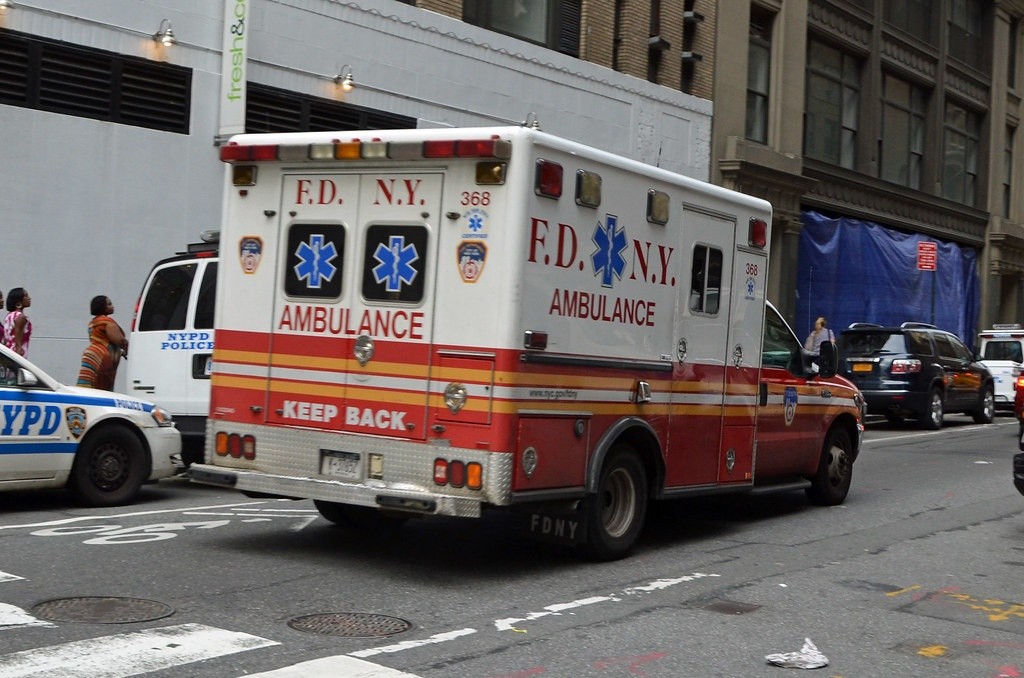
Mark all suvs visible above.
[834,321,995,432]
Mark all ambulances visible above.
[185,120,869,567]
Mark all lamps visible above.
[0,0,17,16]
[333,63,357,93]
[153,18,179,47]
[521,111,543,132]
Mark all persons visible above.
[0,287,32,385]
[806,317,835,352]
[76,296,129,392]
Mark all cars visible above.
[1,345,185,507]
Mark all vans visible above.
[112,231,220,465]
[976,323,1024,412]
